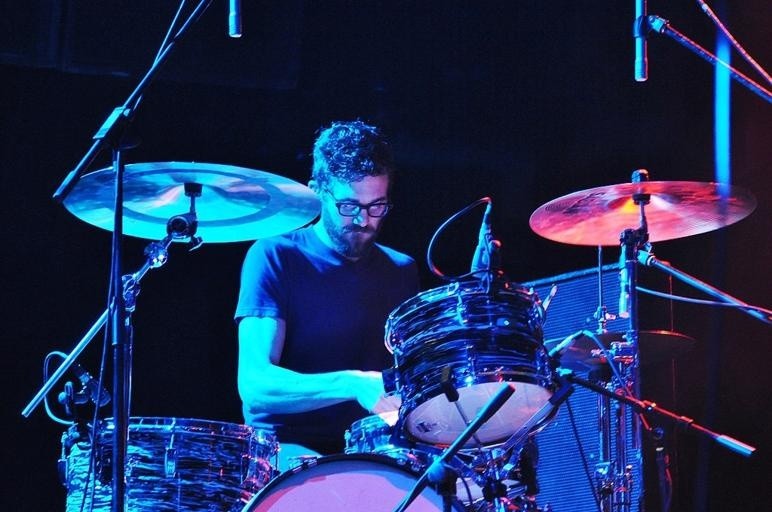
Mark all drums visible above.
[384,281,561,447]
[244,453,464,510]
[343,413,530,505]
[60,417,278,510]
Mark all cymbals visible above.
[530,181,757,246]
[539,331,696,359]
[62,161,322,244]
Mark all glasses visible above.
[321,185,394,218]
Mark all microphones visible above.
[548,330,584,359]
[470,204,492,279]
[634,0,649,83]
[229,0,243,38]
[68,358,111,407]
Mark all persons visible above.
[233,121,421,476]
